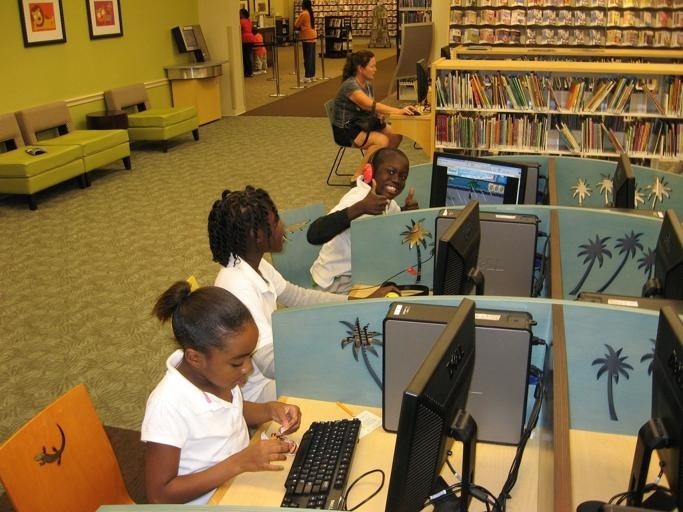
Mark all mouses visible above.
[403,110,420,115]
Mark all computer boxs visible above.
[503,160,539,205]
[433,209,538,297]
[381,302,531,446]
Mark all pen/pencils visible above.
[335,400,356,416]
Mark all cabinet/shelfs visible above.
[294,0,432,64]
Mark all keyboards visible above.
[280,419,360,511]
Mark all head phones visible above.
[380,281,430,297]
[364,148,390,183]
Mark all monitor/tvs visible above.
[430,151,528,207]
[433,200,484,295]
[640,208,683,300]
[384,298,503,511]
[172,25,199,52]
[440,46,452,58]
[605,152,636,209]
[415,58,431,112]
[577,305,683,512]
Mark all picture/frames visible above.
[18,0,67,48]
[86,0,123,41]
[254,0,270,16]
[240,0,249,17]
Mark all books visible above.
[435,57,683,175]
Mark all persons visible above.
[294,0,318,83]
[240,9,255,77]
[140,282,301,504]
[252,27,267,75]
[307,148,419,295]
[333,50,417,187]
[208,185,401,440]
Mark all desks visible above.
[389,103,430,158]
[86,111,129,129]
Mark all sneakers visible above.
[300,76,317,84]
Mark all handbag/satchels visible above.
[353,110,386,132]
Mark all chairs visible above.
[0,383,135,512]
[325,98,365,186]
[15,101,132,187]
[104,82,200,153]
[0,112,86,210]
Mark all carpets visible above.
[244,40,397,116]
